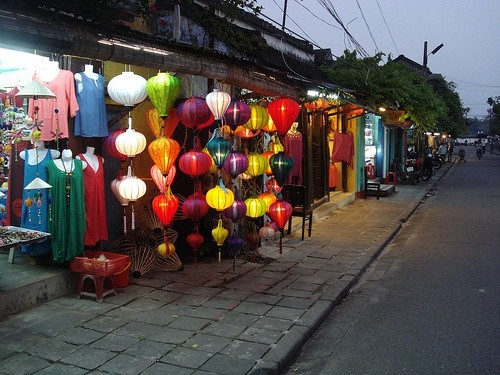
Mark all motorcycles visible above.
[476,148,483,160]
[403,150,446,185]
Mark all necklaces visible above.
[291,130,298,134]
[61,158,74,205]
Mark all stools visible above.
[385,172,397,186]
[77,272,117,302]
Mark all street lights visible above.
[423,41,444,67]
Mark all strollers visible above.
[458,149,466,163]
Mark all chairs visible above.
[363,165,380,201]
[283,184,313,241]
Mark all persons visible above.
[284,122,303,184]
[19,61,108,262]
[438,141,454,162]
[459,140,487,161]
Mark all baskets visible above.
[71,250,128,277]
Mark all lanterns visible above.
[304,98,328,111]
[103,72,300,258]
